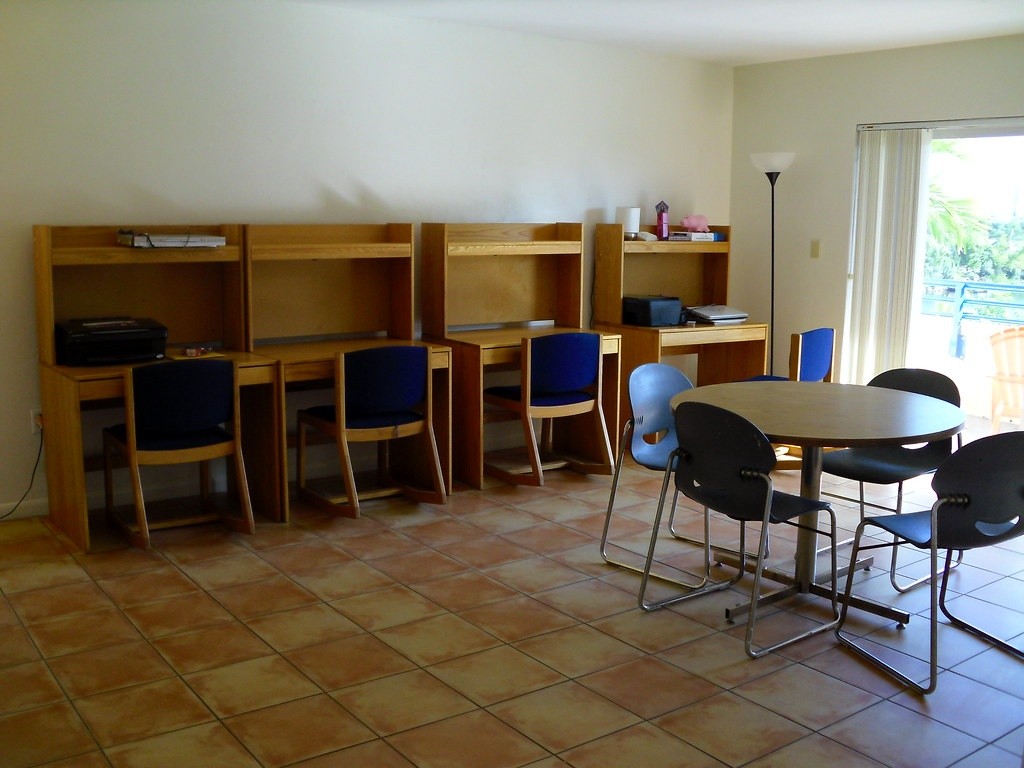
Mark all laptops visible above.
[117,233,227,248]
[685,305,749,320]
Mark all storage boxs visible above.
[624,295,682,327]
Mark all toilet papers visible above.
[615,206,640,233]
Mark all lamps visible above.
[748,151,798,374]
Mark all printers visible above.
[54,317,169,367]
[623,295,683,327]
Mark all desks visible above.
[664,381,966,629]
[31,221,770,556]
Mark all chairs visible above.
[772,327,844,470]
[601,363,1024,695]
[101,356,262,556]
[481,330,620,488]
[988,325,1024,437]
[295,344,447,522]
